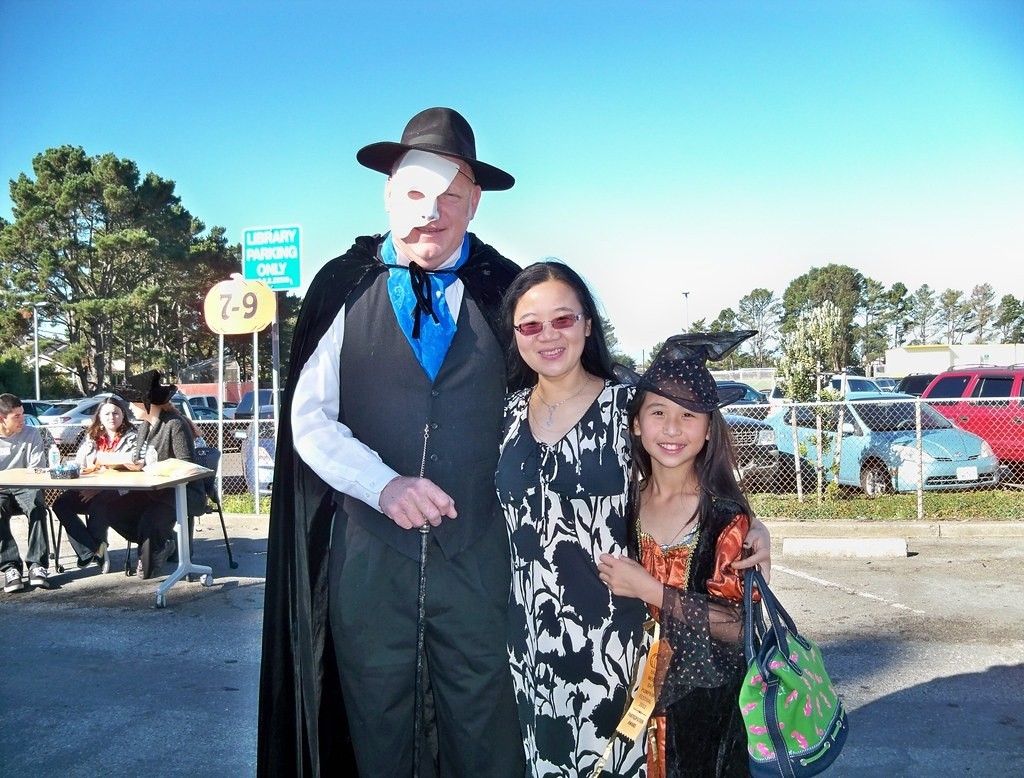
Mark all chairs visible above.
[54,513,132,576]
[11,504,62,572]
[125,447,239,579]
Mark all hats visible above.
[610,330,759,412]
[356,107,515,191]
[111,369,178,415]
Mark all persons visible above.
[257,102,523,778]
[102,368,207,579]
[53,397,138,573]
[598,331,760,778]
[495,262,770,778]
[0,394,50,592]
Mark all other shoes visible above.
[94,542,110,574]
[147,539,176,578]
[77,556,92,568]
[136,544,145,579]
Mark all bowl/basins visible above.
[47,468,80,479]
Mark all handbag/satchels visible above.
[739,566,848,778]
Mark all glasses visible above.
[512,315,582,336]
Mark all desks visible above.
[0,467,215,609]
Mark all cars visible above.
[759,363,1024,481]
[714,381,770,420]
[234,405,276,497]
[20,388,284,453]
[762,392,1000,500]
[718,408,780,492]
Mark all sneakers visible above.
[4,567,24,592]
[28,562,50,589]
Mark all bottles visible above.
[48,444,61,469]
[146,444,158,477]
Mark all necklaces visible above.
[536,372,591,423]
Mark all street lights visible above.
[681,291,691,346]
[22,301,51,400]
